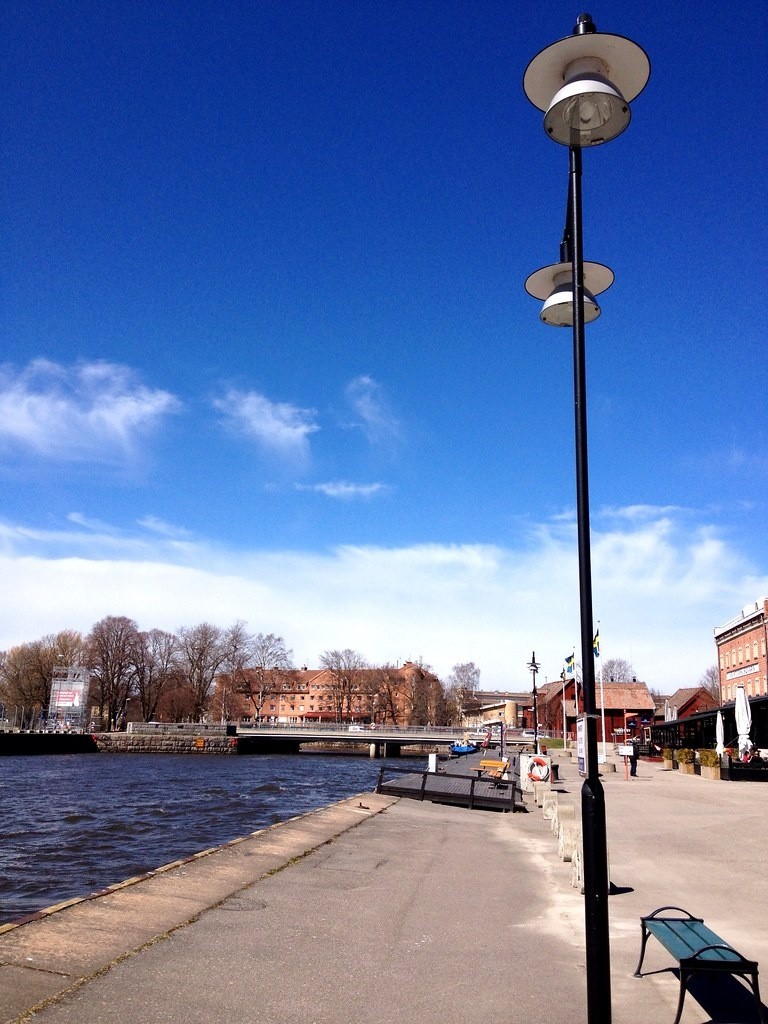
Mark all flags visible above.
[565,653,575,672]
[593,629,600,657]
[560,670,566,683]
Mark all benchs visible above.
[634,906,762,1024]
[470,768,502,779]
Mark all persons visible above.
[0,700,8,721]
[742,750,764,767]
[628,740,640,777]
[116,708,128,731]
[18,707,34,729]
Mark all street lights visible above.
[527,651,539,754]
[522,11,653,1024]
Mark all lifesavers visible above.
[526,757,550,780]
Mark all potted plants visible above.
[698,750,721,779]
[675,748,696,774]
[662,748,676,769]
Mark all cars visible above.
[521,730,551,740]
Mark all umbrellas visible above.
[735,686,752,762]
[665,698,677,722]
[716,711,724,766]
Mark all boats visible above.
[449,740,477,759]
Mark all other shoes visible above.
[632,774,637,777]
